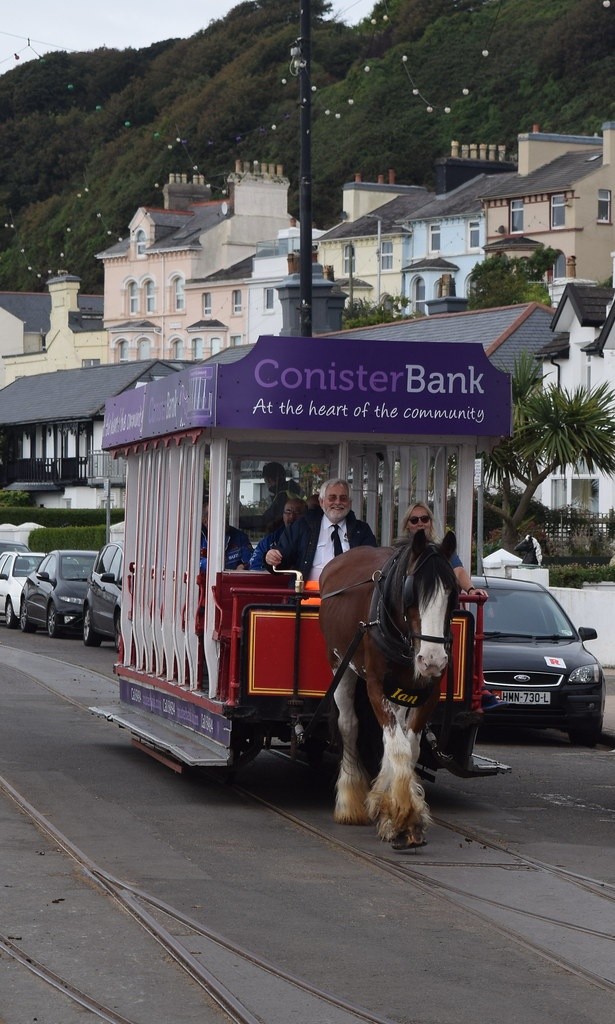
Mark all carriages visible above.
[83,334,515,849]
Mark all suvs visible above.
[80,542,125,656]
[0,540,39,572]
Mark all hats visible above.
[259,462,286,479]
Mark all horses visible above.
[317,528,461,851]
[513,534,543,566]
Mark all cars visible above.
[471,575,606,748]
[17,549,101,639]
[0,550,80,629]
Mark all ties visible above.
[330,525,343,557]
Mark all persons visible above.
[403,500,489,598]
[261,478,379,605]
[200,462,319,571]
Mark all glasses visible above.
[324,495,350,501]
[408,515,430,524]
